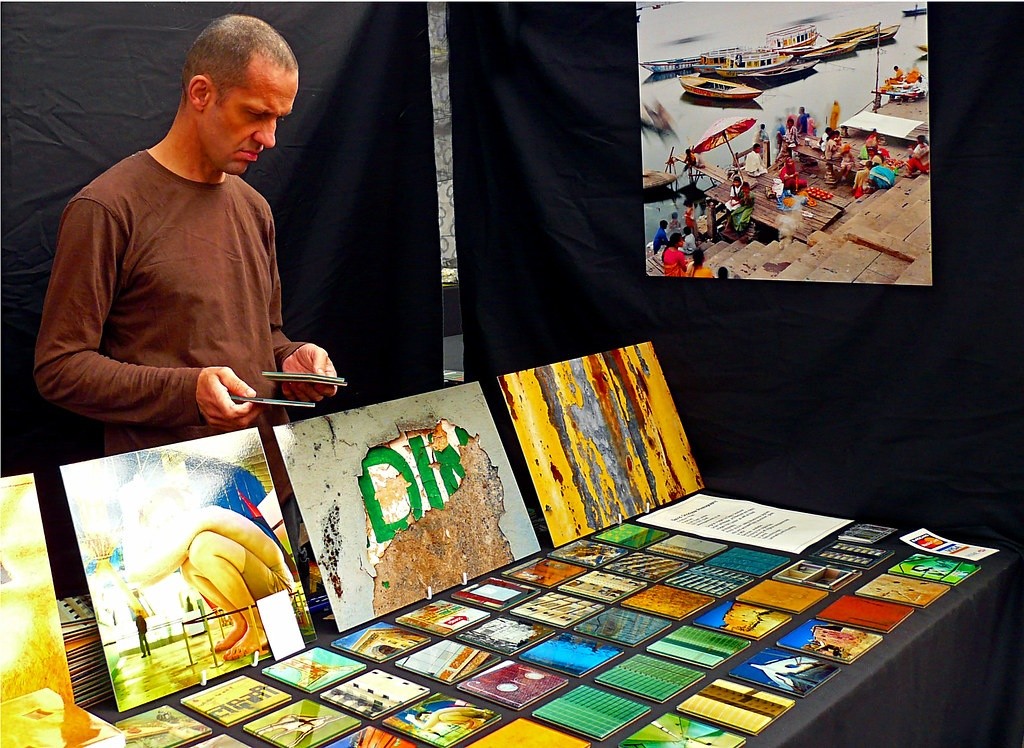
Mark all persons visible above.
[653,65,930,278]
[32,13,347,566]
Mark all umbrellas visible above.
[692,116,758,182]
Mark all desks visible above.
[0,492,1024,748]
